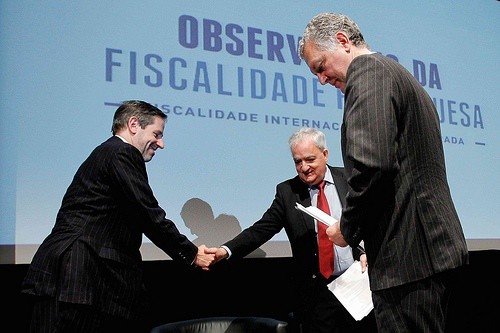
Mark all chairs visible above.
[151,317,288,333]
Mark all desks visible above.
[0,238,500,333]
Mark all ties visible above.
[317,181,335,280]
